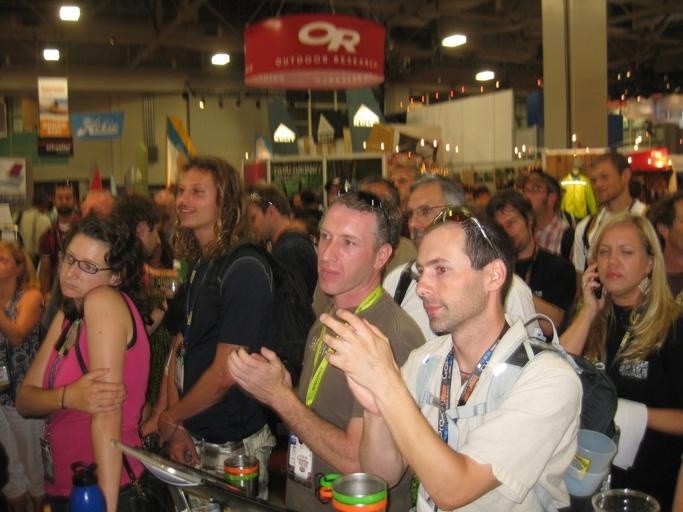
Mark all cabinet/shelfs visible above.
[620,123,677,152]
[452,158,540,196]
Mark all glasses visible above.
[60,250,112,274]
[428,205,500,258]
[356,188,391,243]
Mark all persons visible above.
[0,152,683,512]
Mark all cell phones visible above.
[594,267,603,299]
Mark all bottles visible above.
[66,462,106,511]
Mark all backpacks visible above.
[414,312,618,432]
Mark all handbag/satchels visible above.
[117,467,175,511]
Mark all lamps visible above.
[436,16,467,49]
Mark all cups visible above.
[224,455,259,496]
[316,471,388,512]
[567,429,660,511]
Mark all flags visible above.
[163,113,196,189]
